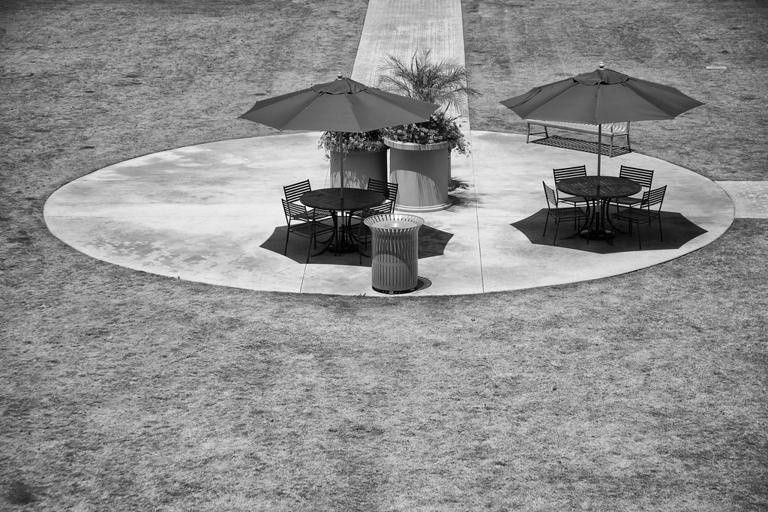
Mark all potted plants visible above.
[319,50,484,211]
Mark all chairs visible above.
[542,164,666,250]
[280,177,397,264]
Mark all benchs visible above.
[527,117,632,158]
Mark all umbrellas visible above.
[498,62,705,232]
[237,71,441,247]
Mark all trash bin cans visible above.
[363,214,424,294]
